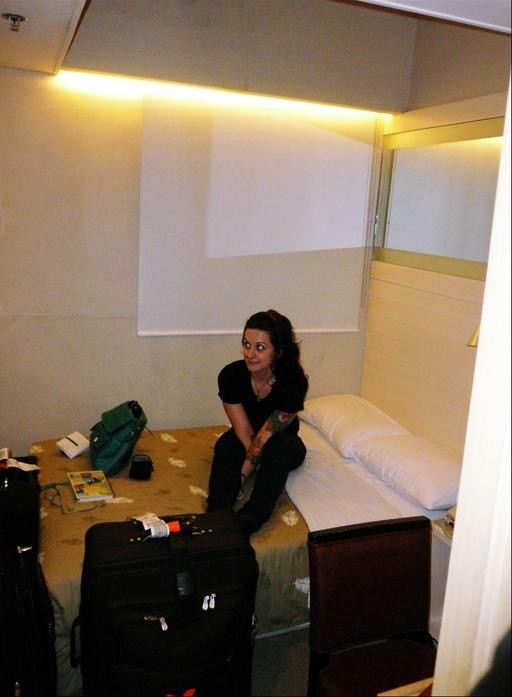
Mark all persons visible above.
[206,309,310,532]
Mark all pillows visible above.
[297,392,412,460]
[353,429,459,511]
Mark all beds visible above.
[28,418,451,697]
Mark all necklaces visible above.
[250,376,272,403]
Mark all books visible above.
[65,470,114,502]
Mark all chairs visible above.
[306,516,436,697]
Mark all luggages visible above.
[69,510,261,697]
[0,447,40,551]
[0,545,57,697]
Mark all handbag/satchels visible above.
[88,400,146,479]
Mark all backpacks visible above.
[89,400,147,477]
